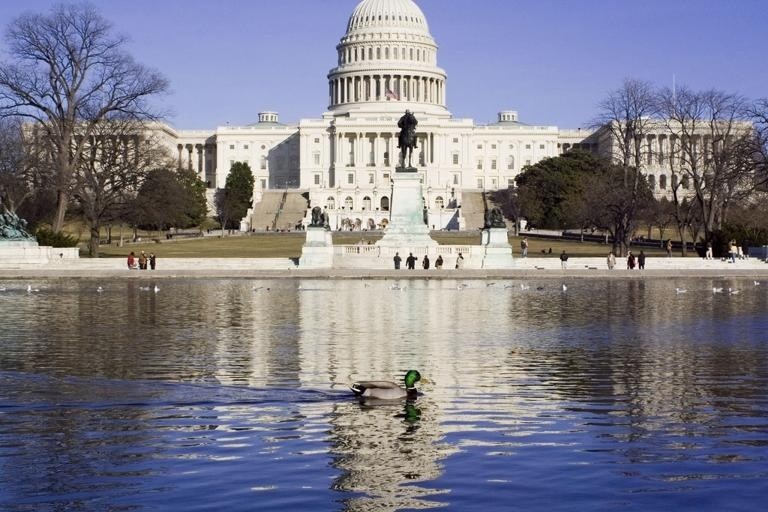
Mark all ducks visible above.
[350,370,431,400]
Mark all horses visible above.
[398,112,415,169]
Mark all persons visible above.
[521,237,528,257]
[423,255,429,269]
[560,250,568,269]
[128,250,155,269]
[397,109,418,148]
[455,253,464,269]
[627,250,645,269]
[394,252,401,269]
[406,253,417,269]
[728,239,743,262]
[666,239,672,257]
[435,255,443,269]
[607,252,616,269]
[704,239,713,260]
